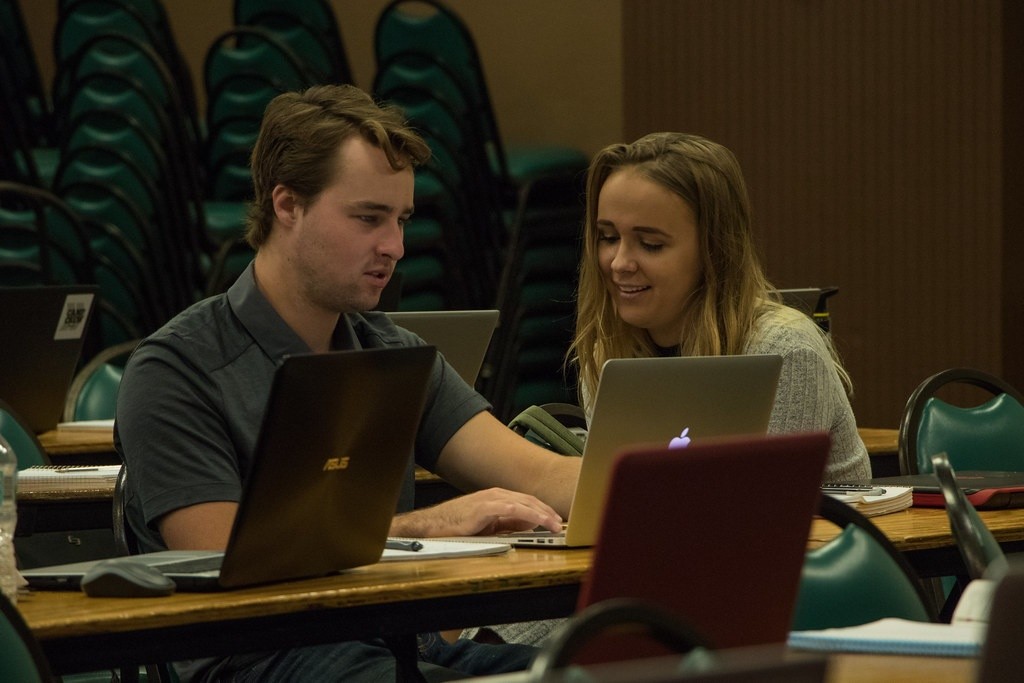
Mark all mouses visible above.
[80,559,176,598]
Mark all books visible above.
[58,419,114,432]
[379,537,512,561]
[17,465,121,490]
[788,617,988,659]
[815,484,914,518]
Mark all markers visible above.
[384,539,425,551]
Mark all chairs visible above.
[789,368,1024,635]
[111,461,181,683]
[0,0,603,471]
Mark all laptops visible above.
[21,288,832,664]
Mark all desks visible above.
[857,425,900,481]
[9,495,1024,683]
[36,421,117,459]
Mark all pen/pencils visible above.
[819,482,888,497]
[54,468,99,473]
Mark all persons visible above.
[114,86,583,683]
[459,133,872,647]
